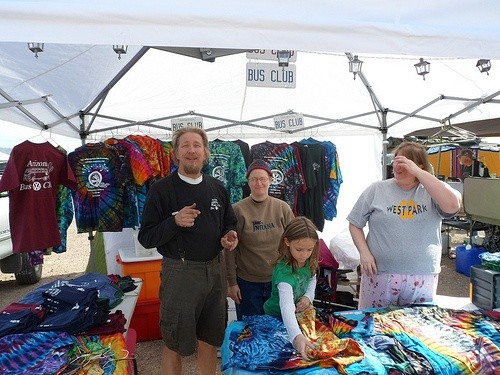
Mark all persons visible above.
[139,127,239,375]
[458,149,492,183]
[229,160,295,323]
[263,217,319,361]
[345,141,462,312]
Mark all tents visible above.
[0,0,500,252]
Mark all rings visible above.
[299,350,304,353]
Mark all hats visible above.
[245,158,273,179]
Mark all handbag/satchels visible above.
[317,238,339,270]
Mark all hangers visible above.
[420,116,478,147]
[26,123,332,145]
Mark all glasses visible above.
[248,176,270,183]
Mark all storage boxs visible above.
[116,246,163,345]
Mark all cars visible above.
[0,160,42,285]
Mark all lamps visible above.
[27,43,493,87]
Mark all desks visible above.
[107,277,144,375]
